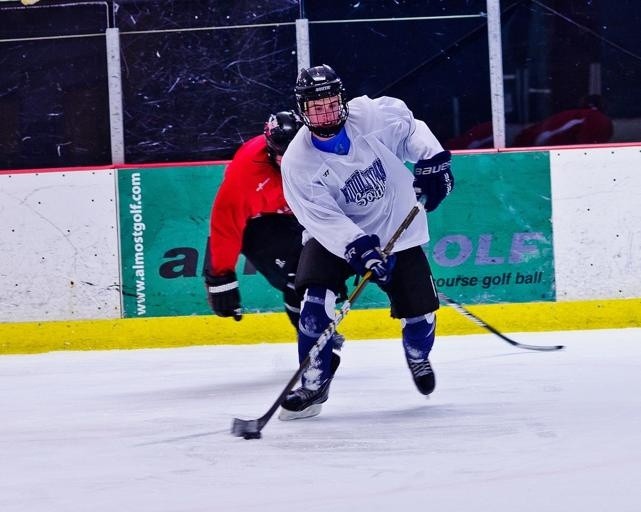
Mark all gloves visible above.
[343,234,396,286]
[412,150,454,213]
[205,269,243,322]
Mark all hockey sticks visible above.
[231,195,428,436]
[435,290,567,350]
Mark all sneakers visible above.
[280,377,332,411]
[407,355,436,395]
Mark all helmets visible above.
[295,62,348,138]
[264,110,304,152]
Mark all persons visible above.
[278,64,454,422]
[204,110,345,359]
